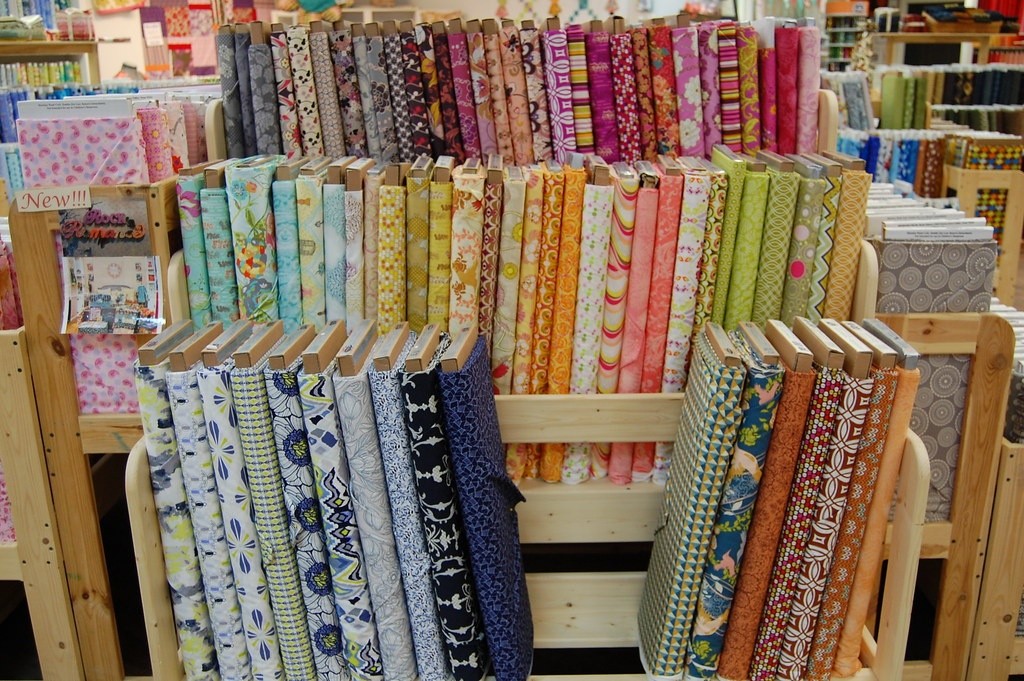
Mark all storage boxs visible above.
[921,11,1004,33]
